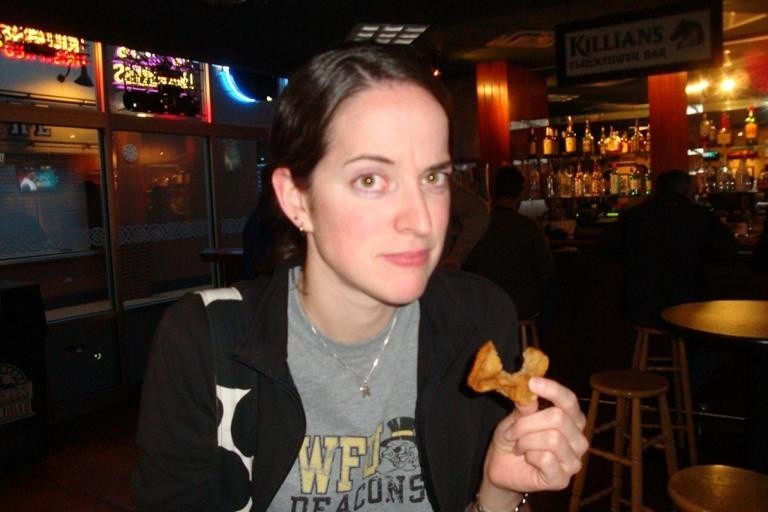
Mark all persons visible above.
[19,167,37,192]
[604,170,740,422]
[462,164,549,318]
[130,42,589,512]
[440,179,492,269]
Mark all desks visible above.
[661,298,768,339]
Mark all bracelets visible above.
[475,491,529,512]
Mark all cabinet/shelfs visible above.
[511,104,768,233]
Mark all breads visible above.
[465,340,549,408]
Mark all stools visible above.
[570,369,679,511]
[625,327,698,467]
[517,320,540,351]
[668,464,767,511]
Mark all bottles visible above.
[519,117,650,196]
[694,107,768,193]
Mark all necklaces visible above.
[293,281,403,397]
